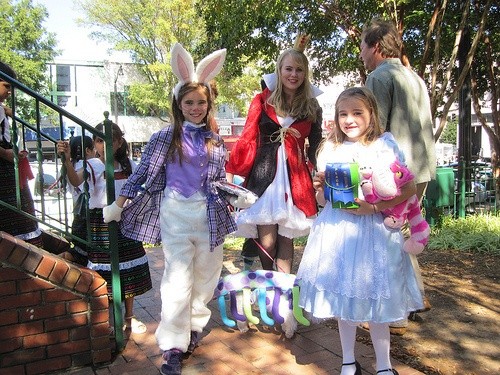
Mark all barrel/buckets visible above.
[425,167,454,208]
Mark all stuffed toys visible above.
[357,133,430,253]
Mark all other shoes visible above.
[360,322,406,335]
[342,359,362,375]
[416,295,431,313]
[377,368,399,375]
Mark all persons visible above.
[103,81,257,375]
[57,121,153,334]
[360,20,438,337]
[68,134,95,264]
[226,51,323,338]
[0,61,45,250]
[295,87,426,375]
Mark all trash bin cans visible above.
[423,167,456,228]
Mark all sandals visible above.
[125,315,146,333]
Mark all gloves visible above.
[103,201,123,223]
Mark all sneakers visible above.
[160,348,183,375]
[187,331,197,352]
